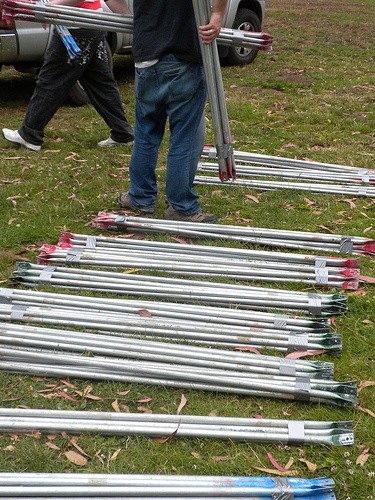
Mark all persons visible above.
[2,0,135,151]
[103,0,229,224]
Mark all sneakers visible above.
[117,191,153,214]
[98,134,135,148]
[163,205,216,223]
[2,127,41,151]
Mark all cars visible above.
[0,0,264,105]
